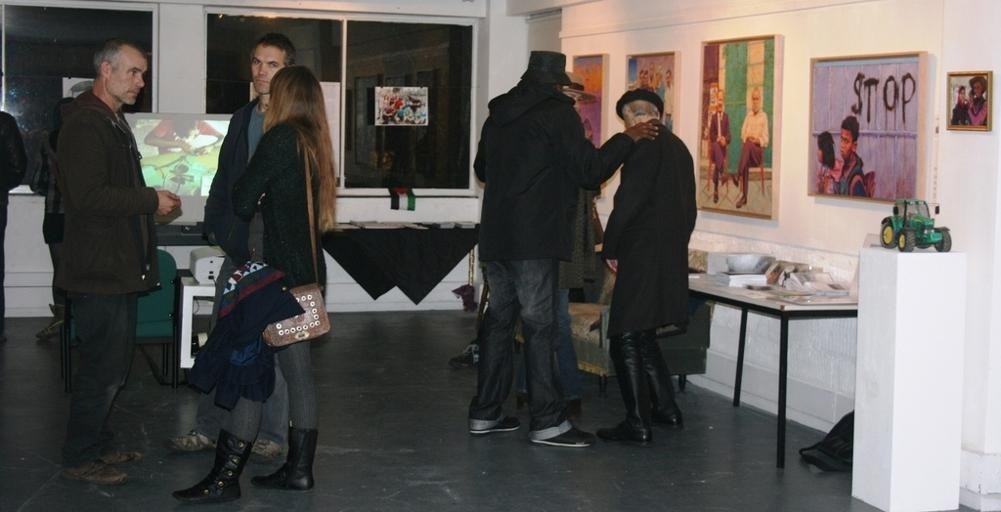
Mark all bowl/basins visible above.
[725,252,763,274]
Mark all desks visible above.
[43,221,482,308]
[684,273,861,467]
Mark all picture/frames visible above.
[572,53,609,199]
[947,71,992,132]
[627,50,683,134]
[807,50,940,209]
[695,34,785,219]
[375,86,428,126]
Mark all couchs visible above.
[479,240,711,400]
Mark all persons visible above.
[559,70,607,303]
[710,90,731,203]
[56,38,182,485]
[817,131,836,194]
[837,116,867,197]
[951,87,971,125]
[175,31,297,458]
[173,66,336,503]
[967,77,987,126]
[0,111,28,344]
[597,88,698,442]
[470,49,596,449]
[733,88,769,208]
[382,94,403,124]
[30,96,74,339]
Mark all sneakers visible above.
[98,450,142,464]
[449,337,481,368]
[62,459,127,485]
[169,431,212,453]
[530,426,594,448]
[251,437,281,464]
[471,416,519,434]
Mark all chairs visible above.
[63,249,179,392]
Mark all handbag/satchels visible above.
[248,123,330,348]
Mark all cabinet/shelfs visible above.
[183,275,216,368]
[855,250,963,512]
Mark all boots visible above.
[251,424,317,490]
[171,428,253,502]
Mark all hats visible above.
[616,88,663,119]
[519,49,572,85]
[562,72,596,102]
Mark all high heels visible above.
[517,394,529,409]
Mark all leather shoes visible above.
[596,421,649,444]
[651,406,683,425]
